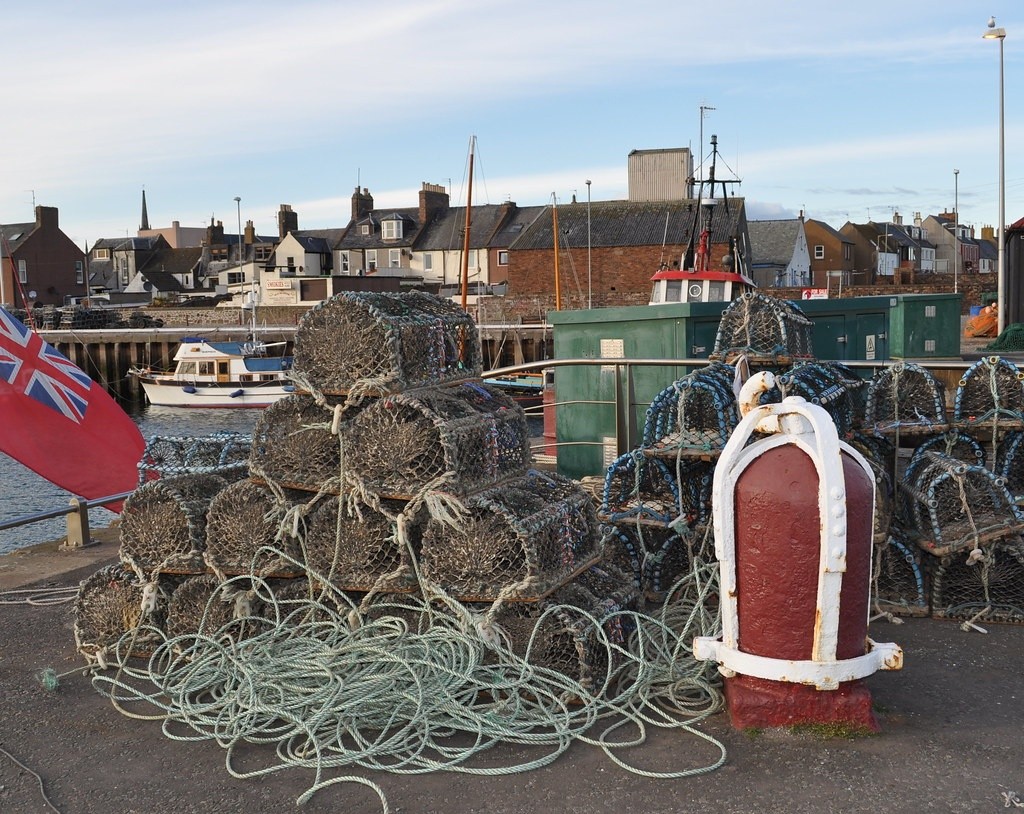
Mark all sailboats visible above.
[439,137,587,411]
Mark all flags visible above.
[0,306,160,515]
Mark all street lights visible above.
[942,223,949,259]
[877,234,894,275]
[235,196,243,322]
[885,222,895,279]
[954,169,959,291]
[981,28,1007,339]
[62,295,71,306]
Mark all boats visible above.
[124,280,297,409]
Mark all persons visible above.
[33,298,43,328]
